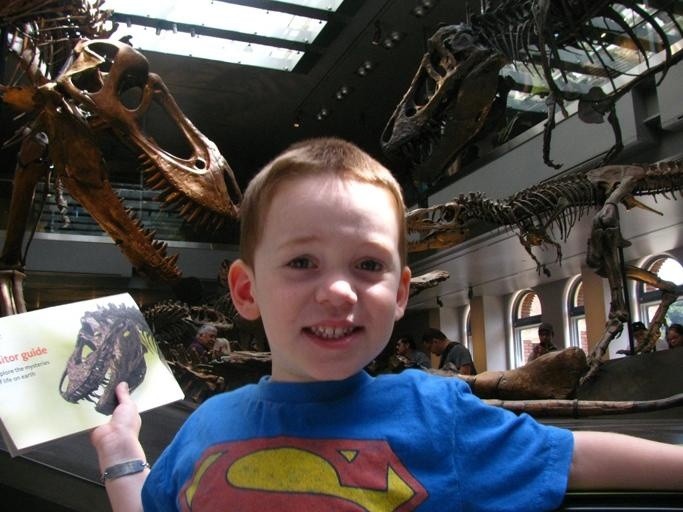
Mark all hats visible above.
[539,323,552,334]
[632,322,647,331]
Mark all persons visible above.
[528,322,561,364]
[421,328,478,375]
[393,335,432,370]
[667,323,683,348]
[187,324,218,356]
[631,321,657,353]
[90,139,683,512]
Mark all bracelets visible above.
[99,459,151,484]
